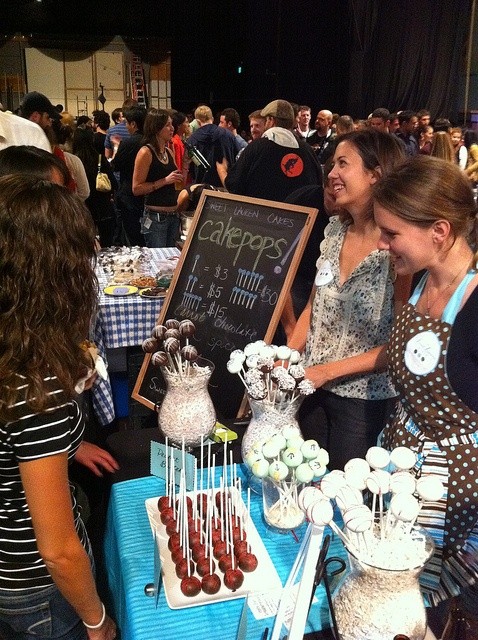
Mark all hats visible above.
[19,91,63,119]
[260,100,294,120]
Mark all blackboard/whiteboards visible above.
[130,188,319,418]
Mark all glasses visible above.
[91,120,99,123]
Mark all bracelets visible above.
[182,164,190,172]
[82,602,107,629]
[152,181,157,192]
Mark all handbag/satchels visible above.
[96,154,112,193]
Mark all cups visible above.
[262,472,308,532]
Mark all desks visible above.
[100,463,430,640]
[92,246,182,429]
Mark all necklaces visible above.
[421,247,474,320]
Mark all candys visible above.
[243,423,329,523]
[155,430,262,600]
[223,337,315,409]
[139,314,201,392]
[297,445,444,564]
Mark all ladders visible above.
[130,53,146,109]
[77,96,88,116]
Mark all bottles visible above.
[181,138,213,176]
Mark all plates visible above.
[139,286,172,299]
[104,285,138,297]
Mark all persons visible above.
[72,124,118,246]
[52,120,91,201]
[0,145,70,186]
[398,110,449,159]
[17,91,63,130]
[372,155,477,626]
[132,108,193,248]
[60,111,74,127]
[75,115,92,128]
[330,114,340,129]
[44,125,75,193]
[112,108,126,125]
[306,109,338,166]
[0,109,52,154]
[295,105,312,143]
[289,103,303,138]
[241,129,247,140]
[104,99,141,244]
[278,162,354,354]
[90,109,111,156]
[272,129,415,473]
[235,109,267,161]
[177,183,214,212]
[171,112,191,189]
[355,108,398,140]
[185,104,243,187]
[109,104,147,247]
[165,108,178,122]
[184,119,200,137]
[187,113,193,123]
[217,108,249,148]
[334,115,355,143]
[449,127,478,183]
[0,172,122,640]
[215,99,323,203]
